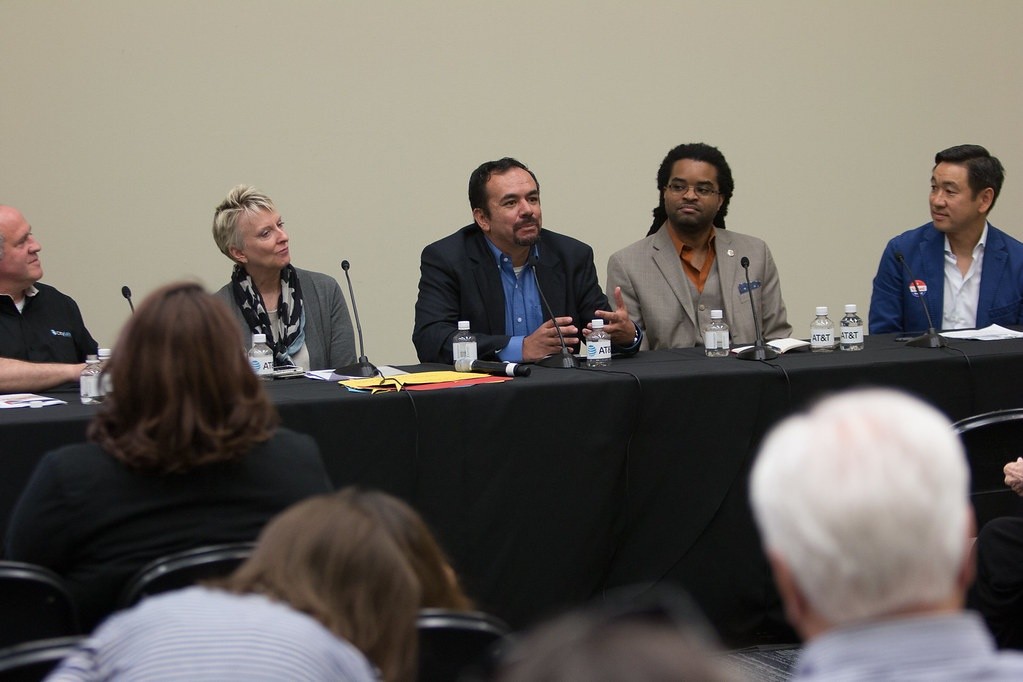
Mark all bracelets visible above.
[634,329,638,345]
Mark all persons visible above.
[411,158,643,363]
[211,186,357,372]
[499,384,1023,682]
[0,279,469,682]
[605,141,792,350]
[869,144,1023,336]
[0,204,100,393]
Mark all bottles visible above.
[97,349,112,392]
[247,333,274,381]
[453,320,477,365]
[586,319,612,367]
[705,309,729,357]
[840,304,864,351]
[80,355,106,405]
[810,307,834,353]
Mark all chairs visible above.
[121,542,257,611]
[414,608,519,682]
[0,562,85,642]
[0,637,85,682]
[949,408,1023,533]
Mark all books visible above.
[0,394,69,410]
[731,337,810,354]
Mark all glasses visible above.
[665,181,721,199]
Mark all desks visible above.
[0,328,1023,644]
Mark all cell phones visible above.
[895,332,926,342]
[273,367,304,376]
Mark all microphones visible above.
[528,256,580,368]
[333,260,378,377]
[455,357,531,377]
[121,285,135,314]
[735,257,779,361]
[894,251,949,349]
